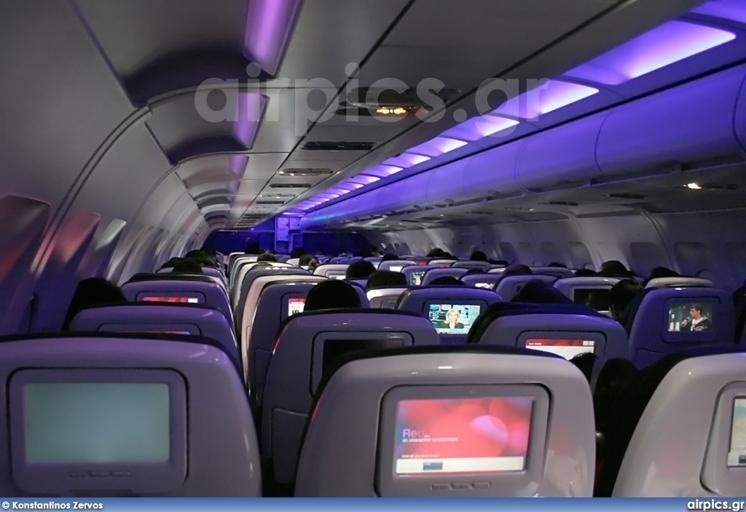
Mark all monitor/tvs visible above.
[7,252,746,497]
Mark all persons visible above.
[63,239,746,498]
